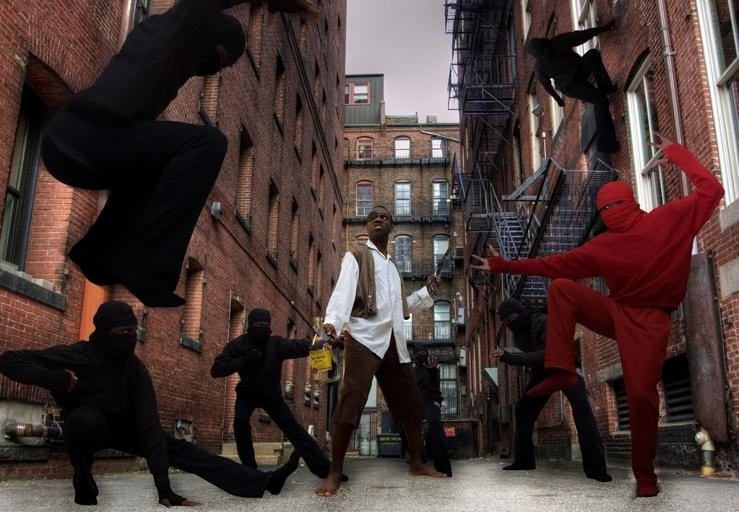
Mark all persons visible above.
[316,205,451,497]
[0,300,302,510]
[208,308,350,486]
[487,297,613,485]
[465,132,725,498]
[40,1,249,309]
[524,15,624,155]
[405,346,453,480]
